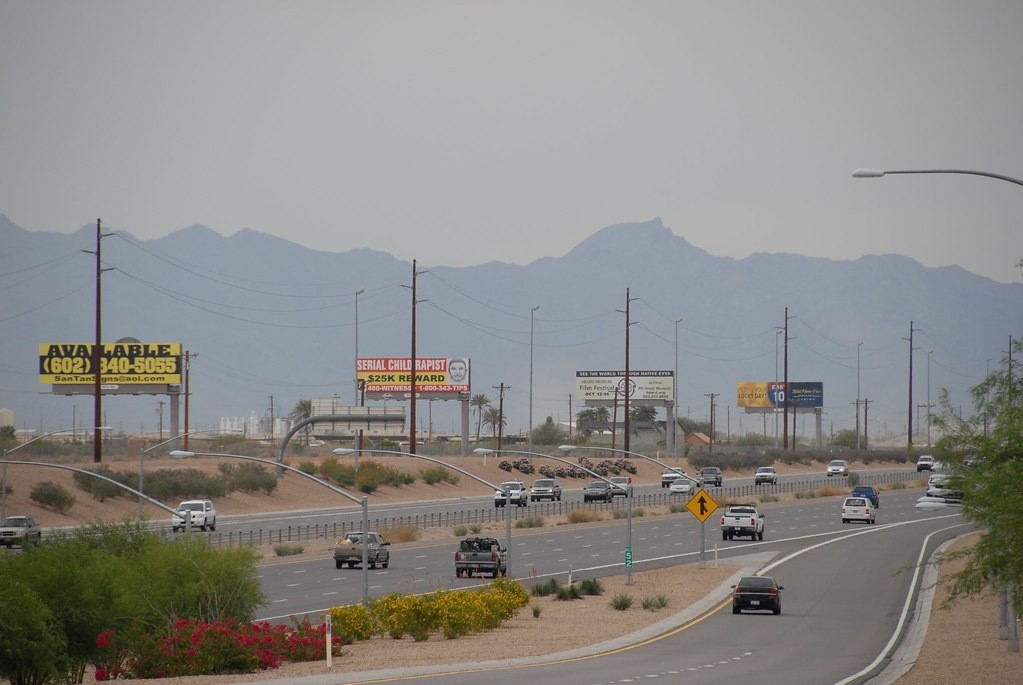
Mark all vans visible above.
[843,485,879,525]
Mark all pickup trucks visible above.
[332,532,391,569]
[721,504,765,541]
[454,538,509,580]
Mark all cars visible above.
[669,479,697,496]
[755,467,778,485]
[827,459,849,476]
[731,576,784,615]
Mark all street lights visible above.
[171,450,368,606]
[333,448,510,579]
[354,288,993,451]
[0,426,244,518]
[559,445,706,567]
[471,449,633,585]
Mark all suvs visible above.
[0,515,41,550]
[495,479,562,508]
[916,455,977,500]
[701,467,722,487]
[661,468,687,487]
[583,477,633,503]
[171,500,217,533]
